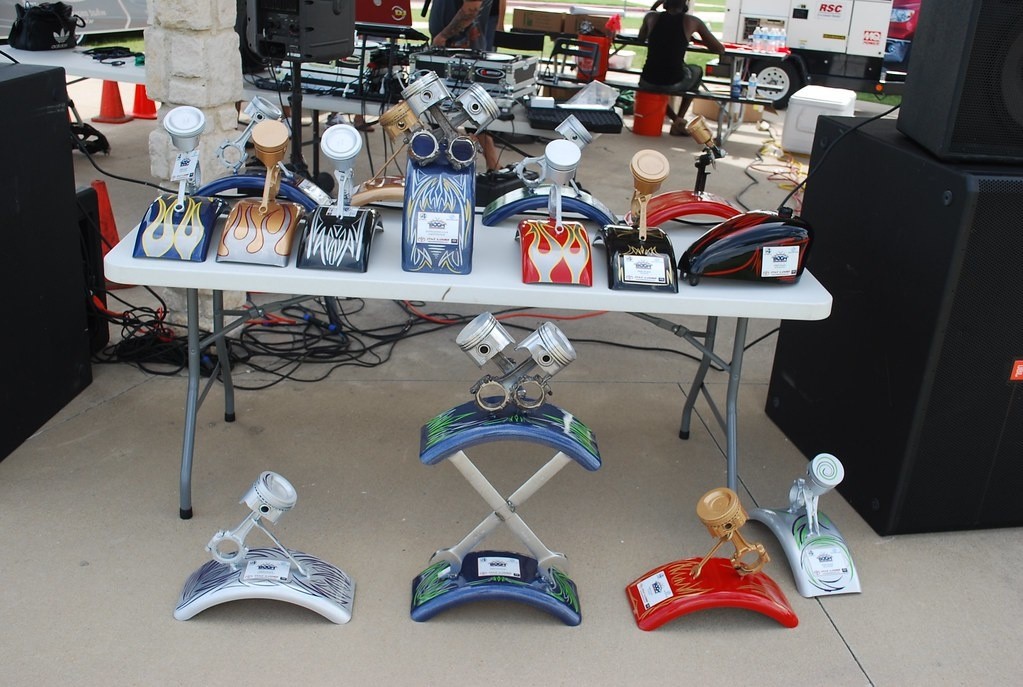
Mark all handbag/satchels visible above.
[8,1,85,52]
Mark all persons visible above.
[637,1,724,137]
[429,0,506,173]
[485,1,505,53]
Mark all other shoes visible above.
[669,119,690,137]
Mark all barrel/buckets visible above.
[576,34,610,83]
[632,91,670,135]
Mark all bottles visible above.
[751,27,787,53]
[731,72,741,99]
[746,73,757,100]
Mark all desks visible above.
[101,204,833,527]
[0,1,790,180]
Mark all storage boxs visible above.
[779,85,857,155]
